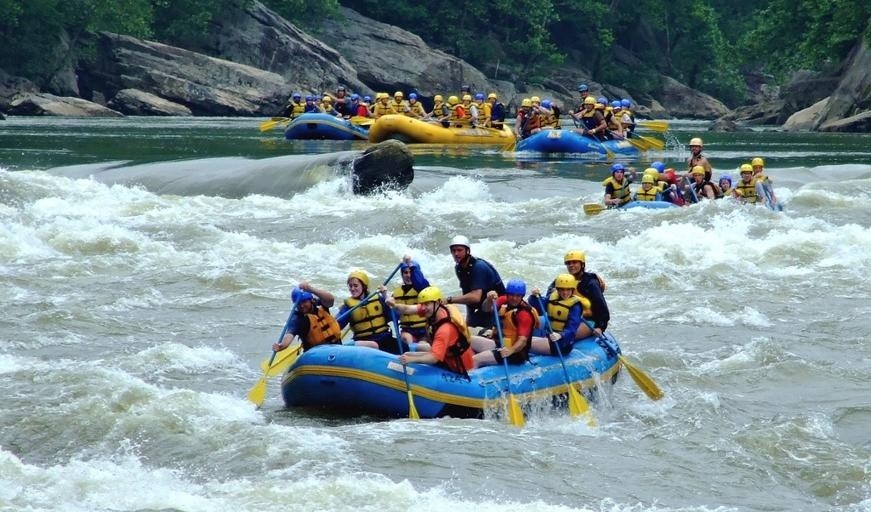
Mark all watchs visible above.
[446,295,453,303]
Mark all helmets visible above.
[363,96,371,102]
[611,100,621,107]
[621,99,630,107]
[555,273,576,290]
[460,85,469,92]
[521,99,531,106]
[530,96,539,102]
[641,174,653,184]
[474,93,483,99]
[739,164,752,176]
[408,93,416,98]
[461,95,470,100]
[447,96,457,104]
[347,271,368,287]
[541,100,549,108]
[719,176,731,187]
[643,168,658,182]
[487,93,496,99]
[375,93,380,99]
[290,287,313,303]
[304,96,312,100]
[401,260,420,272]
[564,250,584,263]
[314,95,321,100]
[689,138,702,147]
[418,286,443,304]
[612,163,623,176]
[692,166,704,176]
[292,93,300,98]
[597,98,607,106]
[578,84,587,92]
[350,94,358,100]
[751,158,762,166]
[322,96,330,102]
[448,235,469,249]
[651,161,663,173]
[380,92,389,100]
[506,279,526,295]
[584,96,594,104]
[394,91,403,97]
[433,95,442,102]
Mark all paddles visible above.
[388,304,420,418]
[491,298,524,427]
[571,112,616,159]
[605,127,650,152]
[623,127,666,151]
[470,122,500,136]
[505,107,533,155]
[351,115,376,125]
[262,290,382,378]
[584,204,625,216]
[634,121,668,133]
[535,293,590,418]
[248,290,305,407]
[260,117,290,132]
[580,315,664,402]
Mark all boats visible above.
[613,195,673,211]
[510,127,637,155]
[369,115,510,142]
[282,110,364,138]
[280,317,622,420]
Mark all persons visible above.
[304,95,322,113]
[663,170,721,206]
[360,96,374,118]
[272,280,343,353]
[323,86,351,117]
[389,253,434,353]
[651,161,666,175]
[446,96,467,128]
[370,93,383,114]
[405,92,430,121]
[550,102,561,130]
[573,96,608,140]
[285,93,308,124]
[719,174,739,202]
[470,279,542,371]
[736,163,769,207]
[602,163,637,208]
[598,97,623,140]
[336,271,398,353]
[532,96,551,132]
[542,99,555,130]
[462,94,478,129]
[634,174,679,204]
[374,93,398,121]
[443,234,505,330]
[575,84,591,114]
[314,93,323,114]
[475,93,491,129]
[350,93,369,117]
[320,96,343,117]
[488,93,506,130]
[528,273,592,356]
[680,136,713,184]
[385,285,476,373]
[611,100,634,137]
[680,165,716,204]
[514,98,542,140]
[752,158,778,211]
[391,91,421,119]
[644,168,679,203]
[621,98,636,132]
[546,249,613,334]
[427,94,450,128]
[460,85,473,95]
